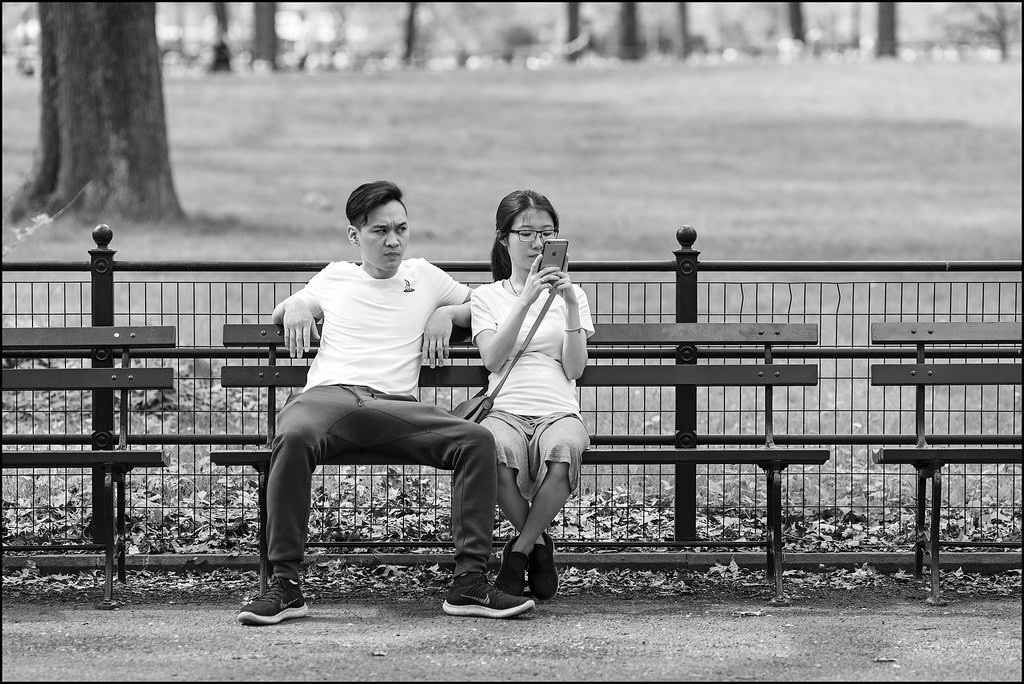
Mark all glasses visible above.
[506,228,559,242]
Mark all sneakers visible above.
[442,575,535,617]
[236,579,309,623]
[526,531,559,601]
[494,534,529,596]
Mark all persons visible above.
[469,188,595,601]
[238,179,536,628]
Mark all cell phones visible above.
[538,239,569,284]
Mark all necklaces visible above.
[508,277,520,298]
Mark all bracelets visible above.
[564,327,583,333]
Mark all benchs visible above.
[1,324,175,608]
[871,321,1021,606]
[210,324,830,604]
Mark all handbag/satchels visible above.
[451,396,494,424]
